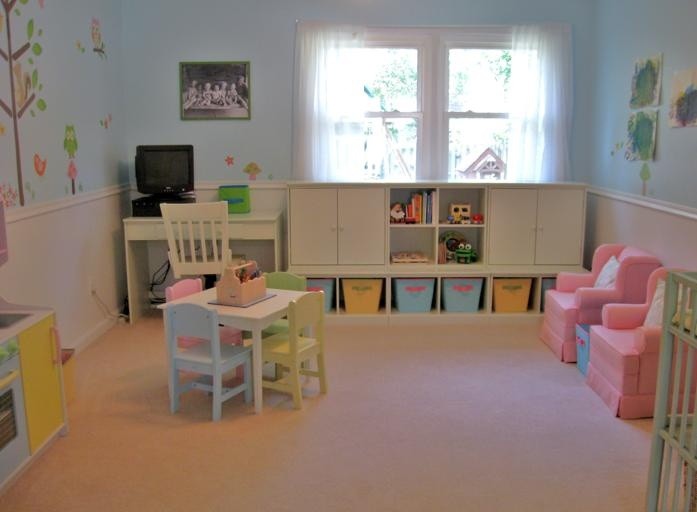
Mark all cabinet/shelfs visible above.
[486,183,587,269]
[386,183,488,268]
[0,297,70,502]
[288,272,585,320]
[288,182,388,267]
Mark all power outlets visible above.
[89,277,98,297]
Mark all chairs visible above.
[155,272,328,420]
[539,243,661,362]
[159,201,242,283]
[586,266,696,419]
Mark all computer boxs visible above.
[131,197,197,217]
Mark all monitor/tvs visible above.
[135,144,194,197]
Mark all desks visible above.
[121,210,284,324]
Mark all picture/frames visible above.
[179,60,251,120]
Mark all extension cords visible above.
[149,303,166,310]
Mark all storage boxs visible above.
[576,321,590,376]
[342,278,383,314]
[541,277,557,312]
[306,278,333,314]
[493,277,533,314]
[442,277,483,313]
[394,278,436,313]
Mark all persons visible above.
[390,201,403,224]
[183,76,248,109]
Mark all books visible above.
[391,251,429,263]
[405,191,437,224]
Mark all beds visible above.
[646,271,697,510]
